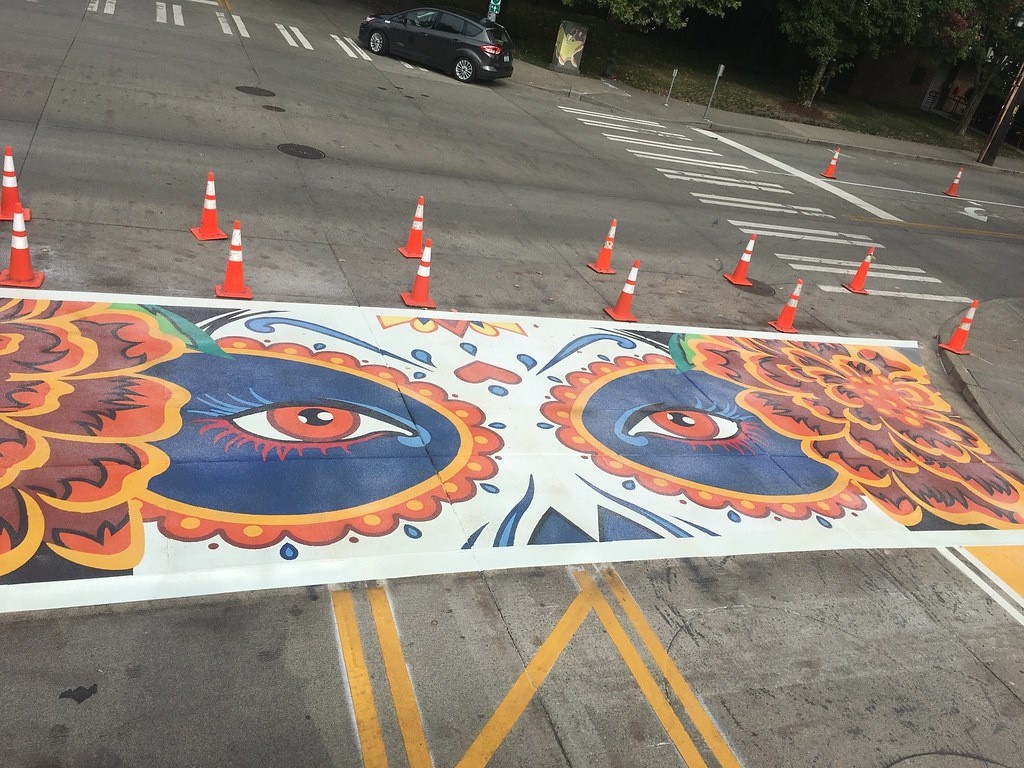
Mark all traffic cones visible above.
[0,201,46,289]
[766,278,804,333]
[400,238,439,310]
[587,218,618,274]
[938,298,981,355]
[0,146,32,220]
[396,194,429,259]
[820,146,841,179]
[215,220,253,300]
[602,257,640,322]
[722,233,757,287]
[942,166,965,198]
[189,171,228,240]
[839,245,876,295]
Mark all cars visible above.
[358,4,516,84]
